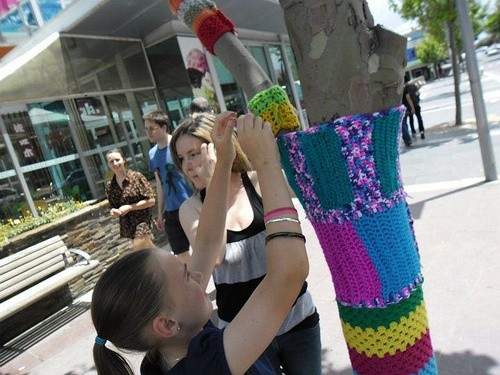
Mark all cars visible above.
[0,189,21,203]
[62,168,90,192]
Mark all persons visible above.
[105,97,237,266]
[90,110,324,375]
[170,113,324,375]
[401,72,426,147]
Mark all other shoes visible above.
[421,133,425,140]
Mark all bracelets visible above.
[264,208,307,247]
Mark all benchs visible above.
[0,235,101,345]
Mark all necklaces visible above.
[167,354,183,363]
